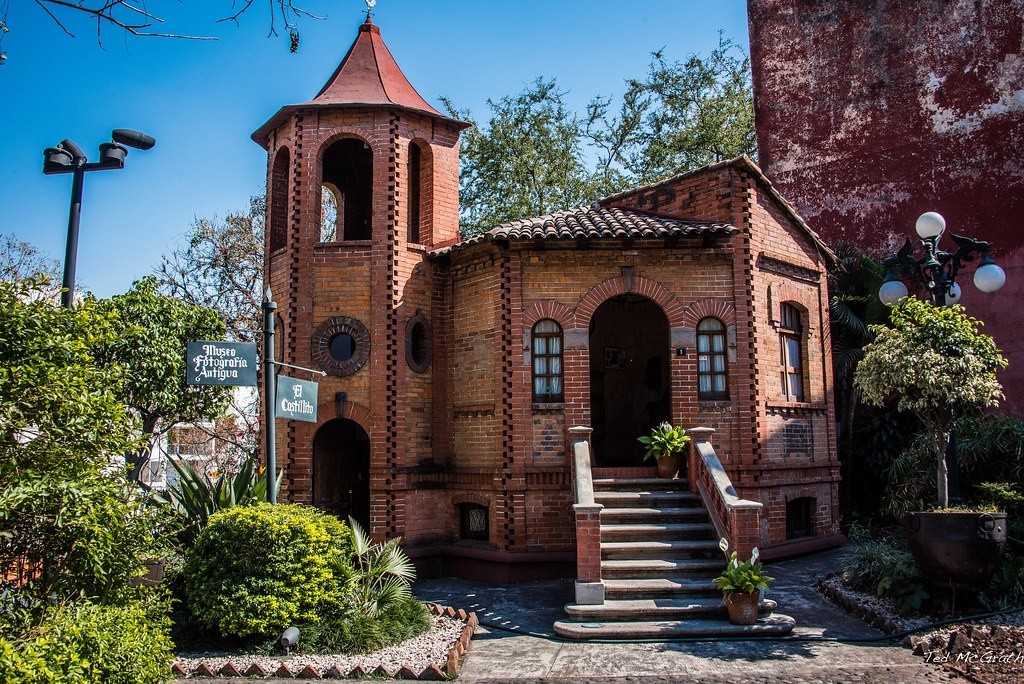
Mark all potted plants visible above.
[902,502,1006,583]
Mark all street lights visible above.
[43,129,156,314]
[879,212,1008,511]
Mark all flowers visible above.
[712,535,777,596]
[638,421,691,463]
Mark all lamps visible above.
[281,626,300,655]
[336,391,348,416]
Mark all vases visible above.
[726,586,761,624]
[657,453,683,477]
